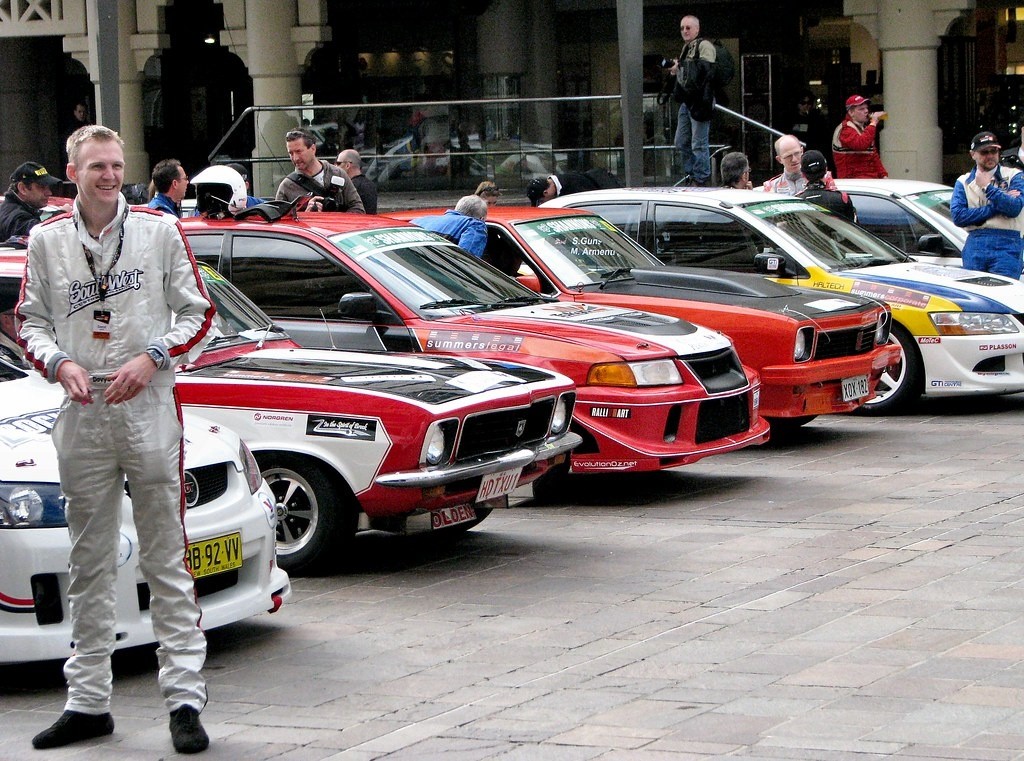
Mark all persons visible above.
[719,151,754,190]
[273,126,366,214]
[785,150,858,257]
[473,181,499,205]
[786,90,831,162]
[60,100,93,181]
[0,161,73,250]
[832,94,889,179]
[147,158,269,219]
[407,194,488,259]
[950,125,1024,281]
[333,148,377,215]
[14,125,220,754]
[0,291,35,382]
[667,15,717,187]
[768,135,838,228]
[526,172,599,207]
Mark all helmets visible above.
[190,164,247,215]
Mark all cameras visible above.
[662,58,682,83]
[294,174,345,212]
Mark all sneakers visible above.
[169,705,208,754]
[32,710,114,748]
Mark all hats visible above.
[970,132,1002,152]
[846,95,869,110]
[10,161,62,186]
[527,179,548,207]
[801,150,827,168]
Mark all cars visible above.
[375,206,901,428]
[179,211,771,476]
[0,327,293,663]
[517,187,1024,415]
[0,245,582,572]
[357,133,571,183]
[754,178,970,270]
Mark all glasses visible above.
[336,161,352,166]
[977,149,1000,155]
[799,100,812,105]
[174,176,188,181]
[285,131,311,140]
[477,186,499,195]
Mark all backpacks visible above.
[583,167,621,190]
[286,159,347,212]
[684,38,735,85]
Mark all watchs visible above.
[145,349,164,369]
[981,183,990,193]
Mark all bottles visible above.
[868,112,889,120]
